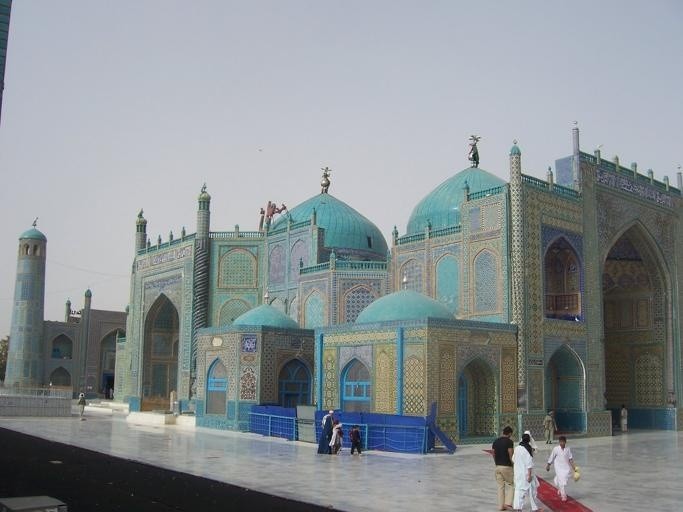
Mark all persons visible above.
[509,433,544,512]
[76,393,86,420]
[491,426,514,511]
[619,403,628,432]
[545,435,576,501]
[328,420,344,455]
[317,409,335,454]
[349,424,362,456]
[519,430,538,454]
[542,410,557,444]
